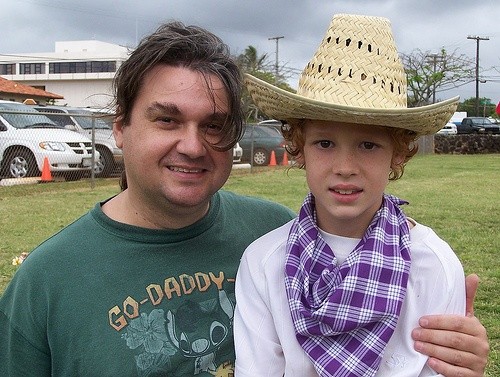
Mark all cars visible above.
[0,99,101,179]
[81,107,117,129]
[232,118,291,167]
[36,105,125,178]
[436,122,457,135]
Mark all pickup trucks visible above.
[452,116,500,135]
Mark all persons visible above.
[0,21,490,377]
[234,14,465,377]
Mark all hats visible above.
[244,14,460,133]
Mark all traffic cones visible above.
[269,150,277,167]
[37,157,55,184]
[282,150,289,167]
